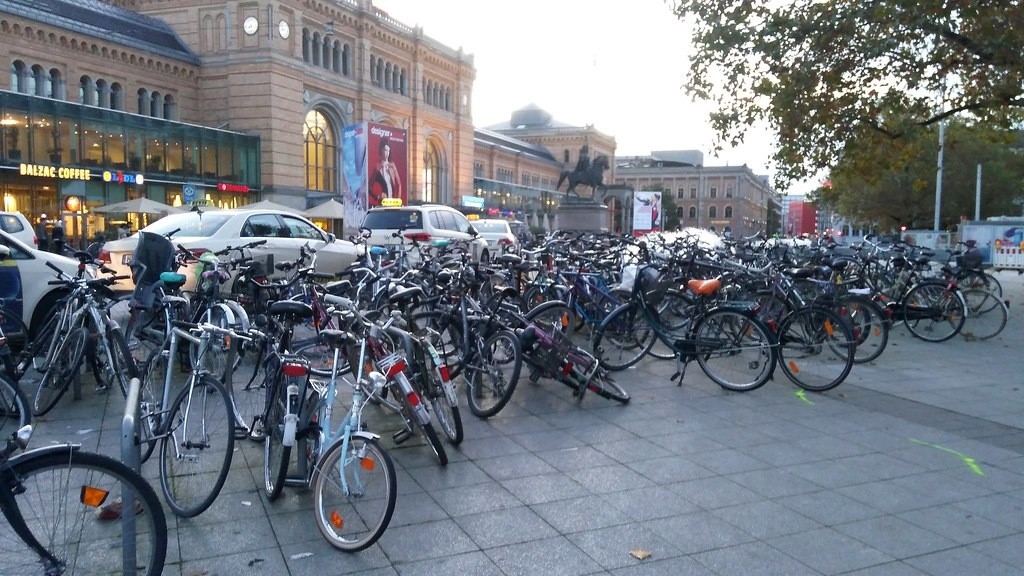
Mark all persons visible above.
[649,194,660,227]
[574,145,593,187]
[52,221,65,255]
[37,218,49,251]
[117,221,132,238]
[368,138,402,204]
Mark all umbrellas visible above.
[91,197,345,236]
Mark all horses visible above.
[556,155,610,205]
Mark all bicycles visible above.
[1,183,1011,575]
